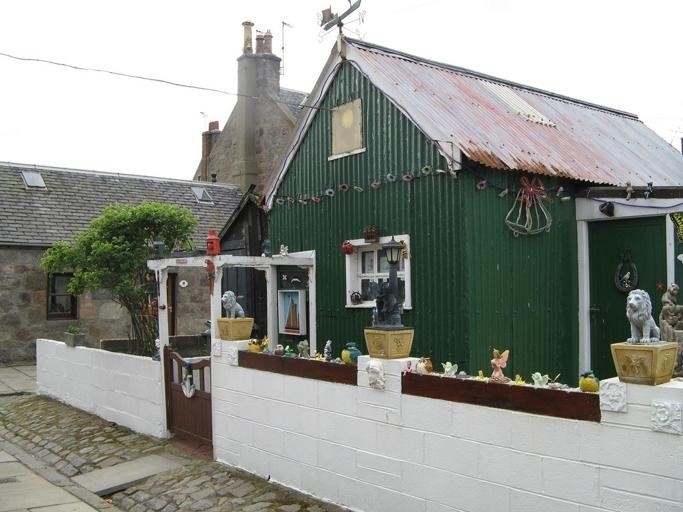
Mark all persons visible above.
[490,348,510,378]
[417,357,427,374]
[441,361,459,377]
[531,372,549,387]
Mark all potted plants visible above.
[62,325,86,347]
